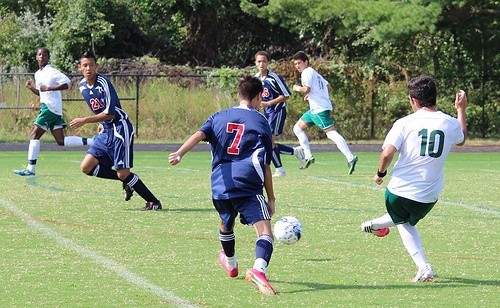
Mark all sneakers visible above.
[217,248,237,278]
[412,264,436,285]
[123,182,133,201]
[348,155,358,175]
[14,170,35,177]
[272,168,286,177]
[140,200,162,211]
[91,135,98,147]
[361,223,389,237]
[300,157,315,169]
[244,268,275,297]
[295,146,304,166]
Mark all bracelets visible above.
[377,167,387,178]
[296,85,301,92]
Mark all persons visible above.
[69,52,162,211]
[361,74,467,284]
[253,51,305,177]
[290,51,358,175]
[169,75,276,295]
[14,48,98,176]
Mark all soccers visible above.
[273,216,302,245]
